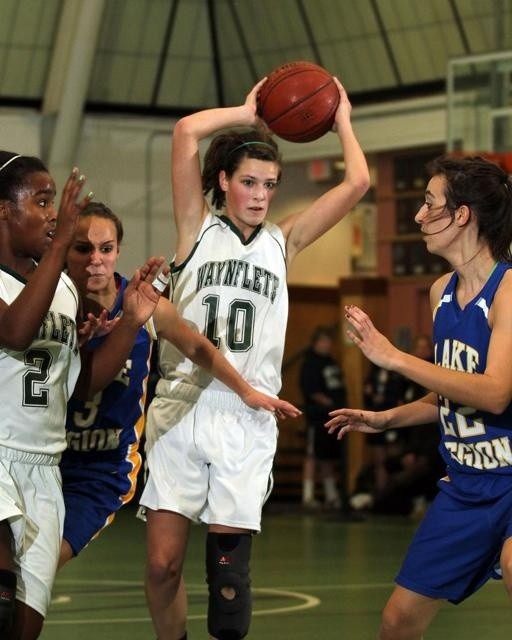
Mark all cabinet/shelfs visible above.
[375,142,467,284]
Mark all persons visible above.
[297,322,442,517]
[2,200,307,600]
[135,75,371,639]
[323,152,512,638]
[1,143,176,638]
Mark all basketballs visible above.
[259,63,339,142]
